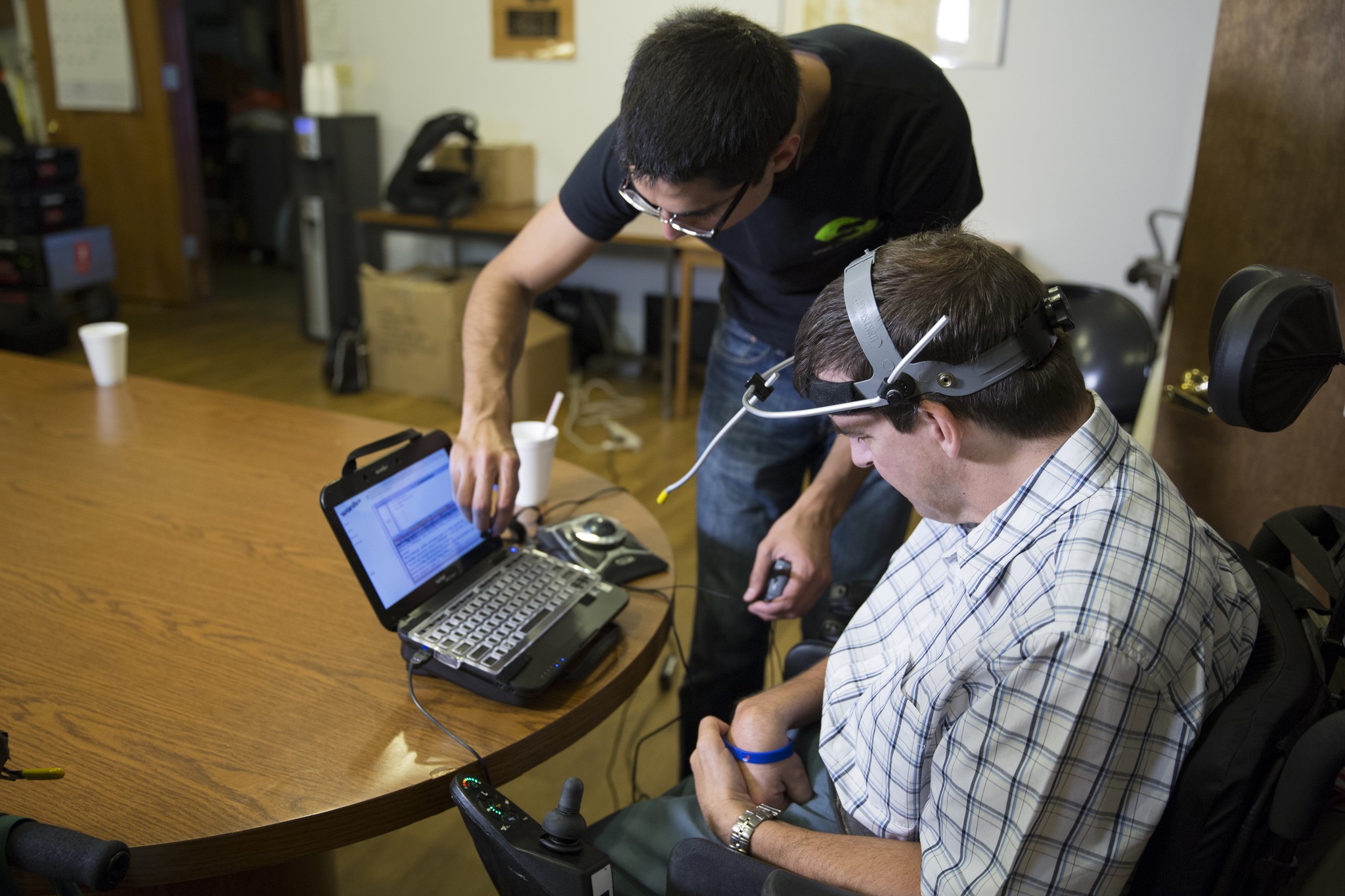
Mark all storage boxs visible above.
[362,261,486,403]
[433,147,535,213]
[512,317,575,418]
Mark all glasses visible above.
[617,164,751,238]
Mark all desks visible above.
[359,199,735,415]
[2,341,673,894]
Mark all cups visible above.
[78,321,129,388]
[511,421,561,508]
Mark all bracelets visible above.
[721,738,793,763]
[729,803,782,853]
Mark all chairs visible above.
[666,503,1345,896]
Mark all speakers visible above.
[302,110,382,342]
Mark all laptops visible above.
[318,426,630,691]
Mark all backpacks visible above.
[385,109,482,219]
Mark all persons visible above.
[449,11,982,777]
[596,235,1256,896]
